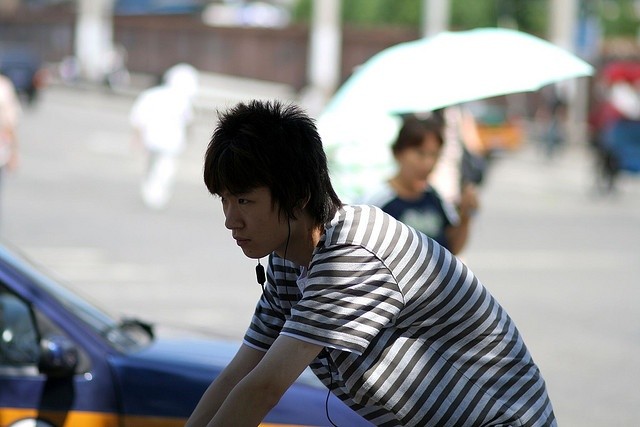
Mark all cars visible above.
[0,243,379,426]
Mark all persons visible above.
[363,119,481,254]
[129,62,197,211]
[0,74,23,228]
[184,100,560,426]
[398,103,487,201]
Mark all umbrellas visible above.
[311,25,596,150]
